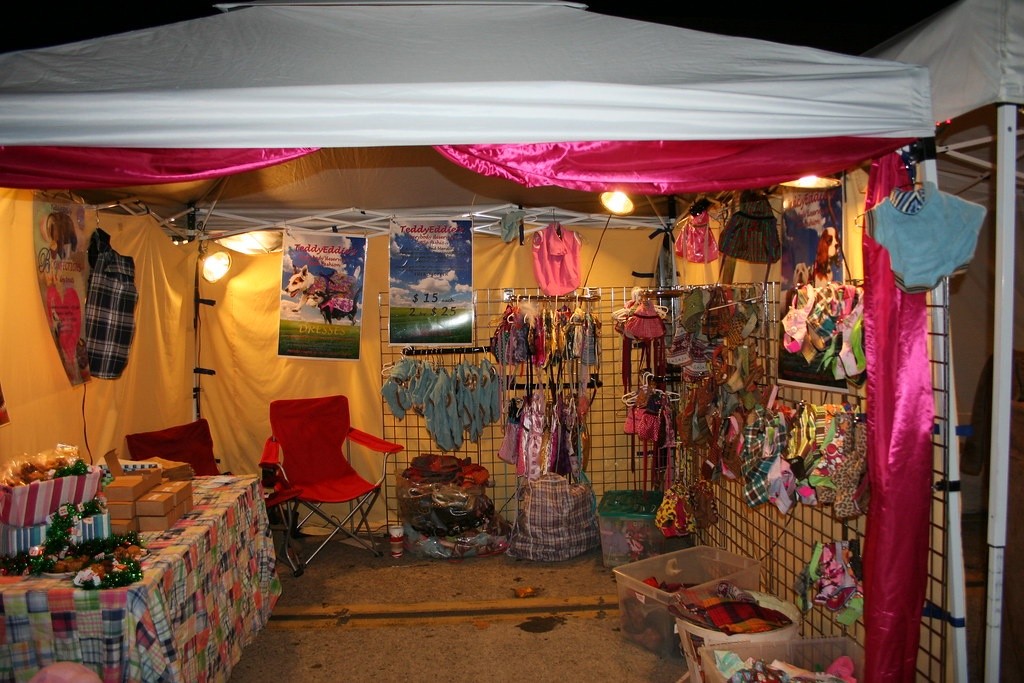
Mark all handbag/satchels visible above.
[509,473,602,561]
[498,420,520,466]
[691,439,718,529]
[655,439,697,538]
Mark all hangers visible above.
[508,380,597,417]
[854,164,922,228]
[505,294,592,324]
[94,205,105,229]
[379,346,496,385]
[621,371,680,405]
[551,210,562,231]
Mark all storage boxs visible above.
[613,547,761,659]
[596,489,690,568]
[0,450,194,555]
[696,637,864,683]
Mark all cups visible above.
[389,526,405,558]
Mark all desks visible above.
[0,475,279,683]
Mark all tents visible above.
[0,0,1024,683]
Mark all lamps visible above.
[190,249,233,468]
[781,176,840,188]
[599,190,634,215]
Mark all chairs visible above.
[258,396,404,577]
[126,419,220,477]
